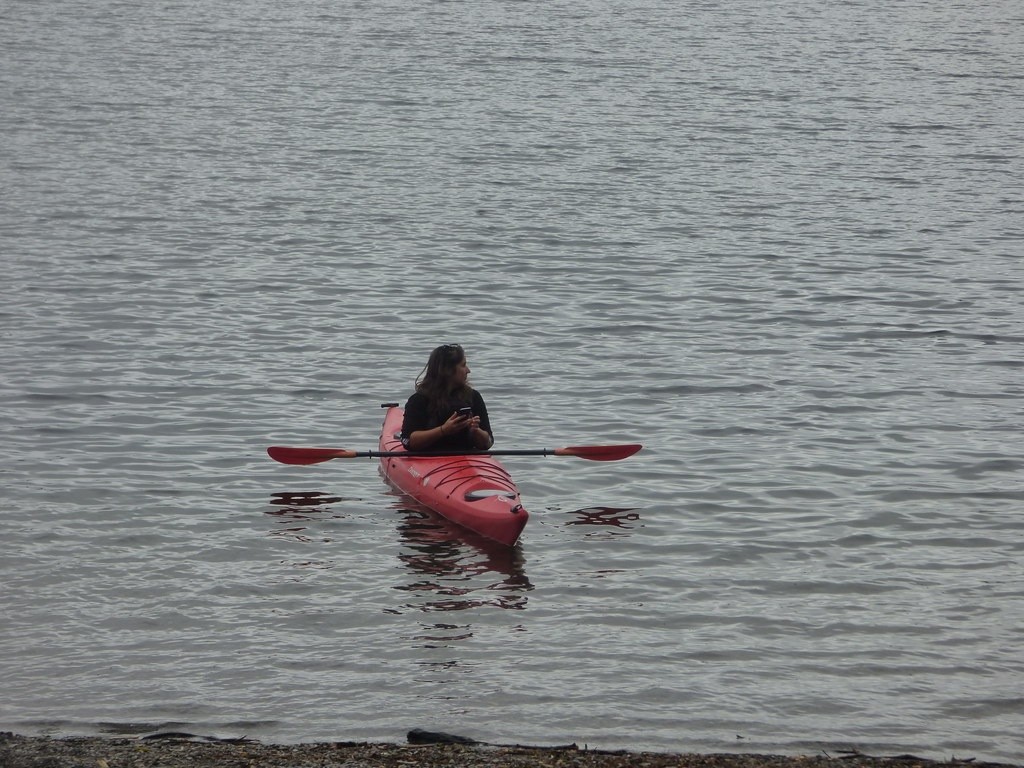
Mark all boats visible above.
[379,402,529,548]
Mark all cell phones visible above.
[460,407,471,420]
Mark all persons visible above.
[400,344,495,452]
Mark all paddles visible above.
[267,444,641,467]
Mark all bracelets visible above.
[441,426,444,436]
